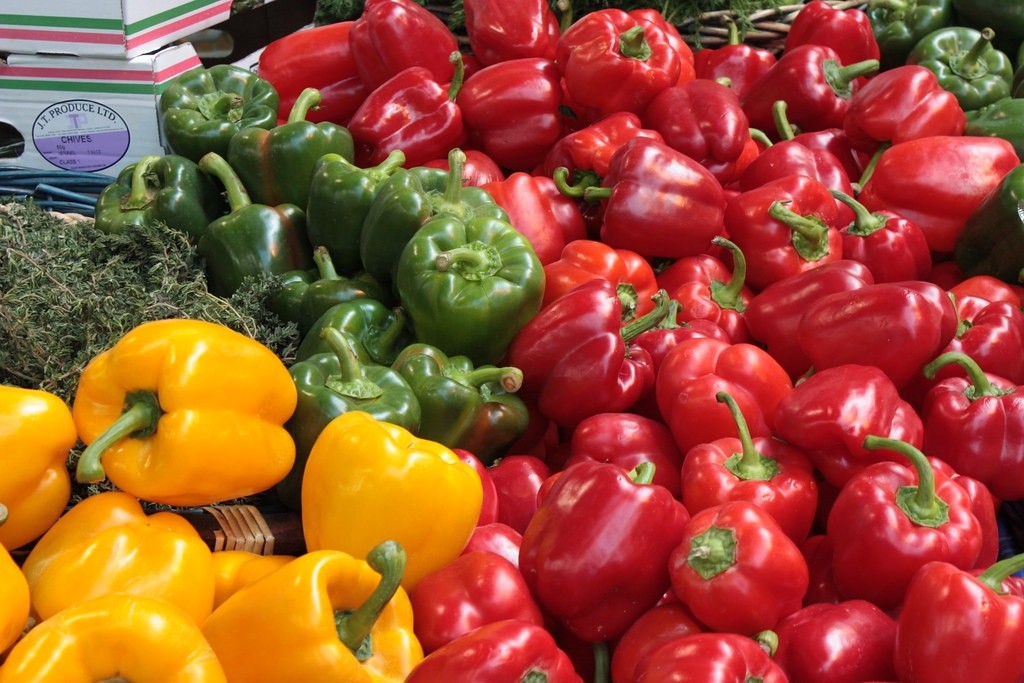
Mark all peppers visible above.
[0,0,1024,683]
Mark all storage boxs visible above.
[0,42,205,178]
[0,0,232,60]
[183,0,318,73]
